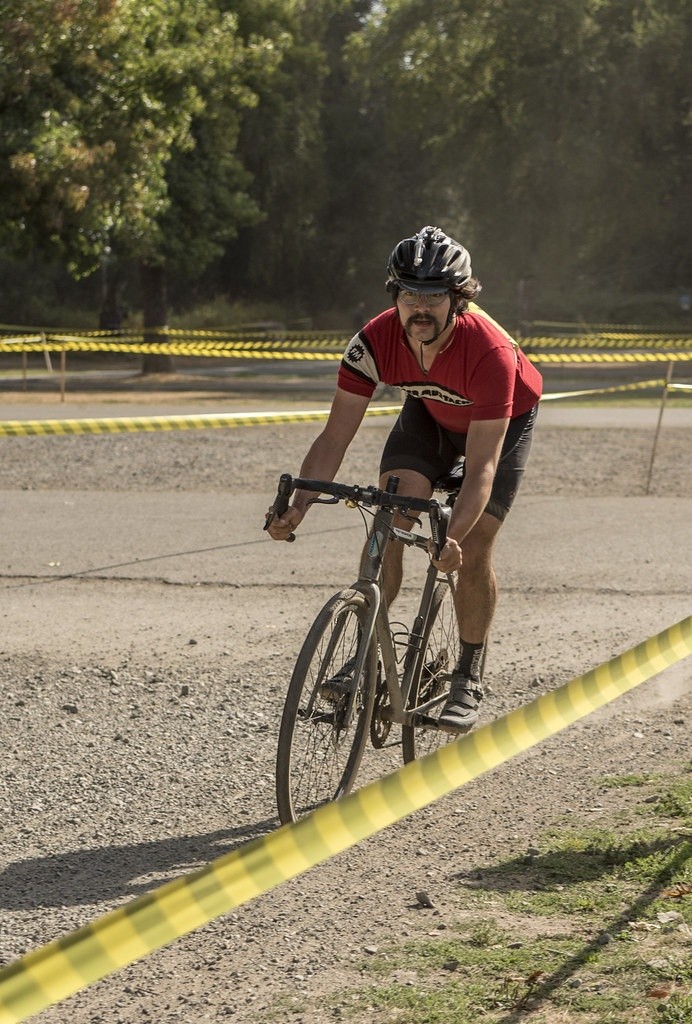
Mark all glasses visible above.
[398,289,448,305]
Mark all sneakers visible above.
[436,668,484,729]
[318,656,382,694]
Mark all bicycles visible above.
[262,452,486,825]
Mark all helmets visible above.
[384,225,473,294]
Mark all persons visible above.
[265,226,543,730]
